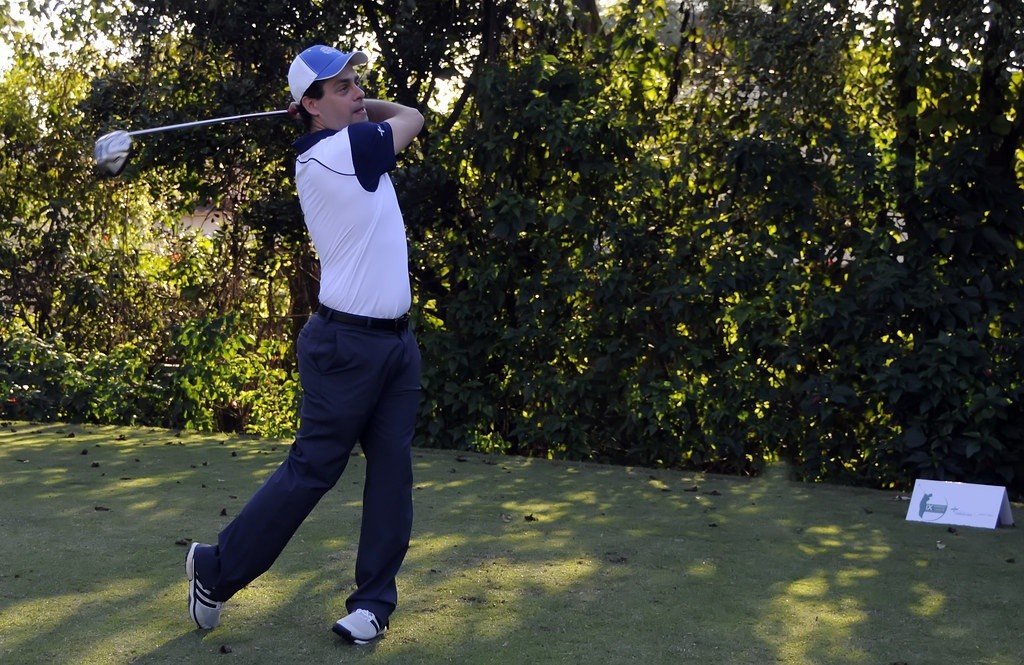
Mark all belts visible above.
[318,305,409,332]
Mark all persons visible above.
[185,45,425,643]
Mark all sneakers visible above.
[332,609,387,645]
[186,542,223,630]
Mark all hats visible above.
[288,45,368,104]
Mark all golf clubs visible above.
[94,109,290,176]
